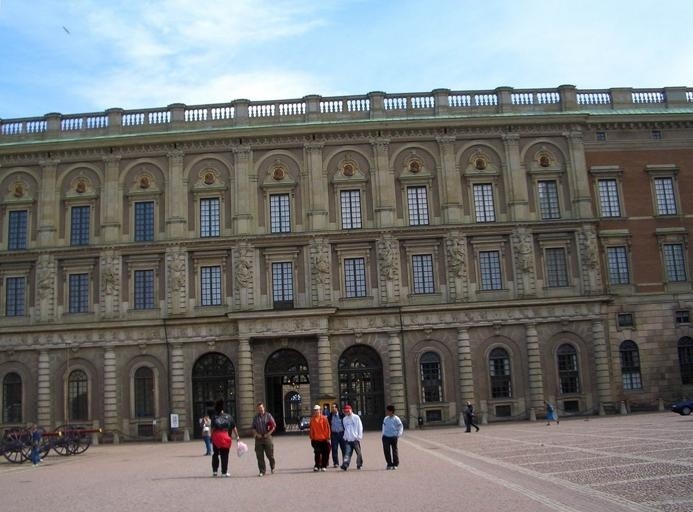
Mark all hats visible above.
[313,405,320,409]
[344,405,351,411]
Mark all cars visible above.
[299,415,311,430]
[671,397,693,415]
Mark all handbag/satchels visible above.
[266,423,275,431]
[212,431,231,448]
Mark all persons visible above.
[543,400,559,426]
[210,404,240,477]
[31,423,41,466]
[251,402,277,477]
[327,403,346,468]
[199,414,212,456]
[309,404,331,472]
[464,400,480,433]
[339,405,364,471]
[381,405,403,470]
